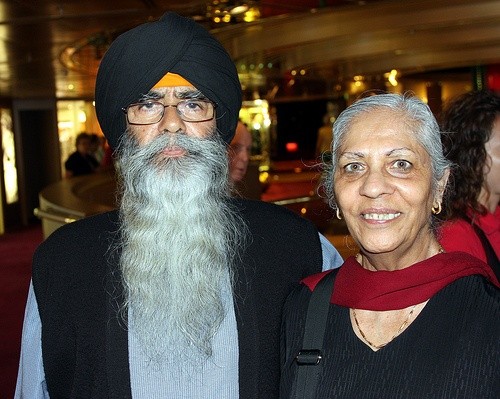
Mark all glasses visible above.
[121,99,216,126]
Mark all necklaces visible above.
[352,248,445,349]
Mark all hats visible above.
[94,12,242,146]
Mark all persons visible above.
[13,11,345,399]
[279,93,500,399]
[431,90,500,283]
